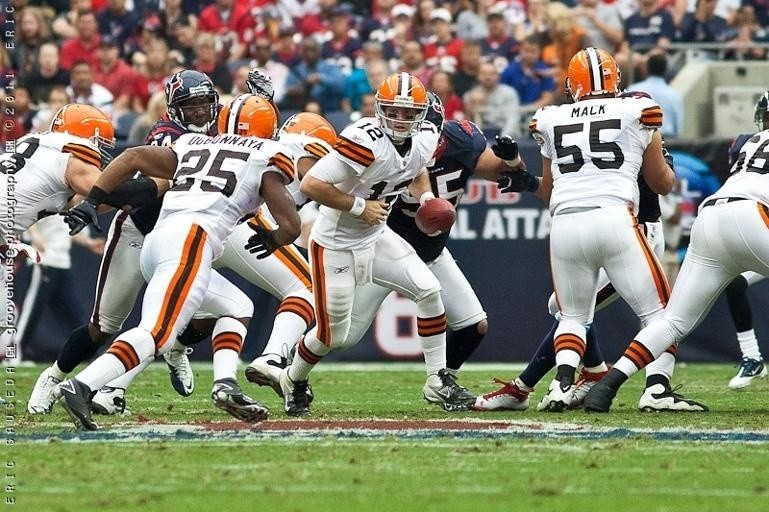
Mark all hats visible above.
[390,3,415,19]
[429,8,452,23]
[485,6,503,19]
[99,34,119,49]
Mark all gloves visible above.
[491,134,522,167]
[58,198,103,236]
[494,168,540,194]
[244,221,281,259]
[245,68,276,102]
[418,191,445,237]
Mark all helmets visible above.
[164,69,220,136]
[216,92,281,141]
[752,90,769,131]
[564,46,625,103]
[48,102,117,172]
[373,70,430,143]
[279,111,337,159]
[425,89,445,131]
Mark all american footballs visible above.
[415,198,457,233]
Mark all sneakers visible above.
[210,377,270,423]
[243,352,315,417]
[162,345,196,398]
[728,356,768,390]
[536,359,618,412]
[420,367,534,414]
[27,365,129,434]
[637,382,709,413]
